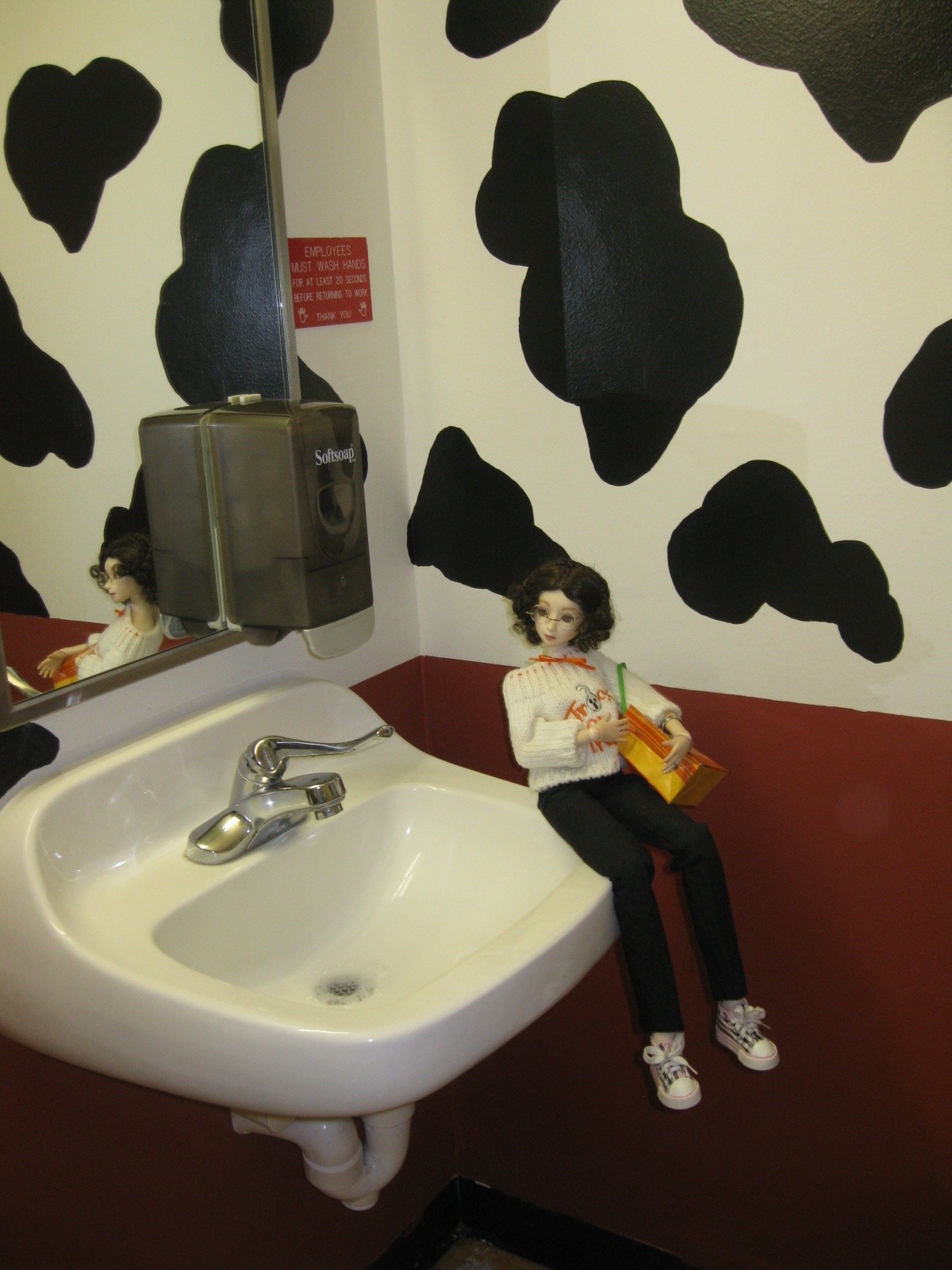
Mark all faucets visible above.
[182,726,395,866]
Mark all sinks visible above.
[0,679,633,1118]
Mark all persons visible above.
[38,533,164,680]
[503,560,779,1109]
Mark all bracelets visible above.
[662,715,682,731]
